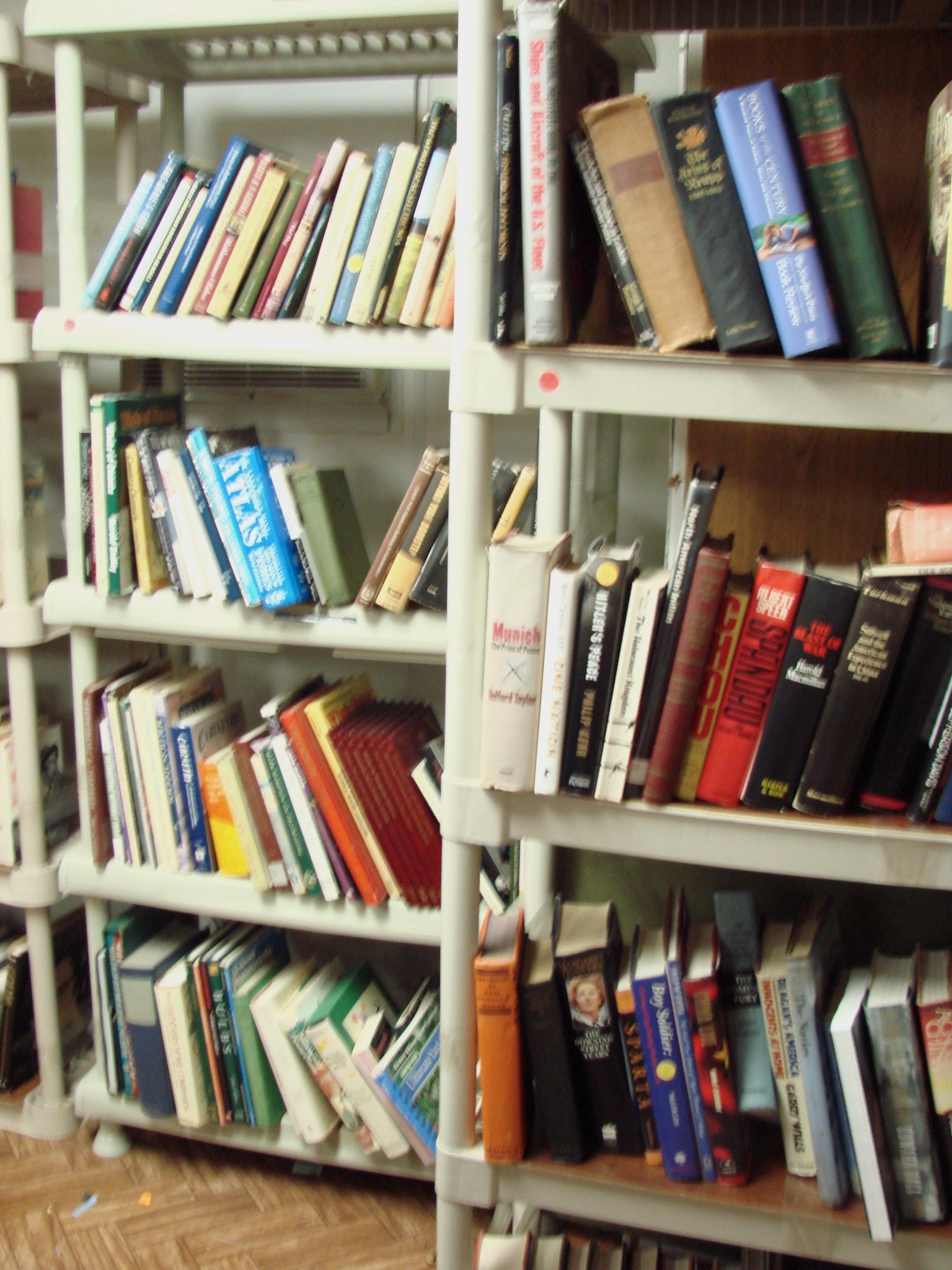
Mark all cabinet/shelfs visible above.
[0,0,951,1269]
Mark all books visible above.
[2,14,952,915]
[0,886,952,1270]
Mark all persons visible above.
[570,979,612,1025]
